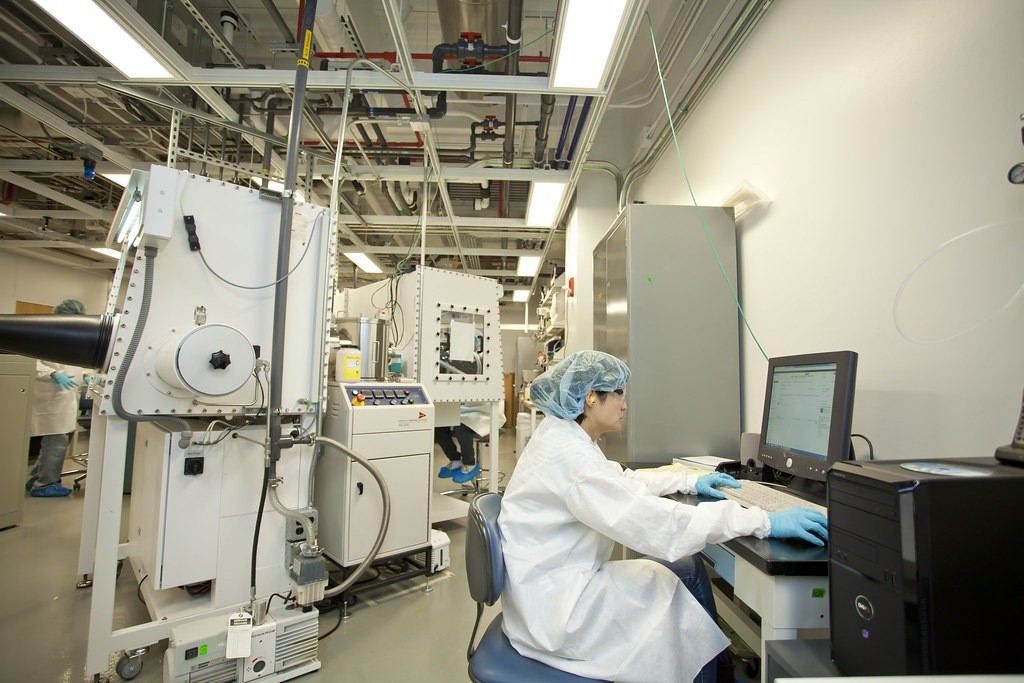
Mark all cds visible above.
[902,462,992,476]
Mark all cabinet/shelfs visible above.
[0,355,37,529]
[513,268,564,437]
[593,204,740,461]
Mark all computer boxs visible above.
[826,456,1024,676]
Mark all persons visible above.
[27,298,91,497]
[498,350,828,682]
[436,330,506,483]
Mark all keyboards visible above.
[715,478,828,520]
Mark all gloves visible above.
[769,505,828,545]
[697,474,740,498]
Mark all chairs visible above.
[466,493,615,683]
[56,398,94,490]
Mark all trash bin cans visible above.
[516,412,544,461]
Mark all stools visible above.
[440,428,506,496]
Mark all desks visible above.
[620,461,830,683]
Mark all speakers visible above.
[740,433,764,474]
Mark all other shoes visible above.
[445,460,461,469]
[460,463,475,474]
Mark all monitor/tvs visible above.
[757,350,857,511]
